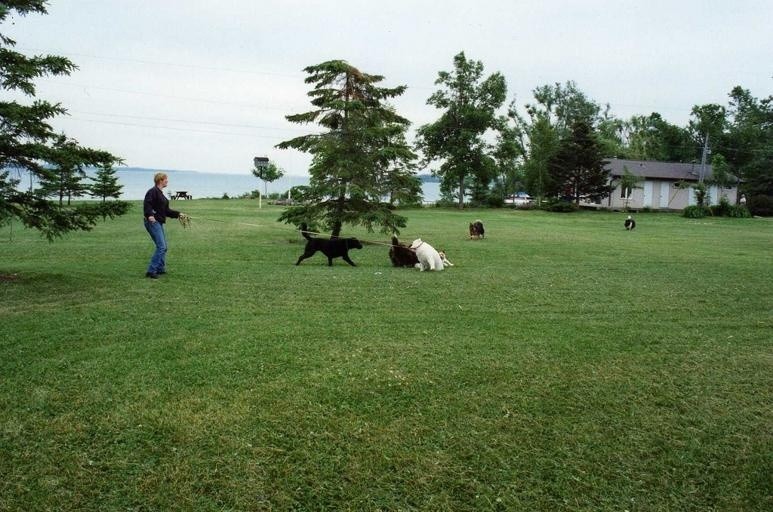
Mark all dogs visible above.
[296,221,363,267]
[469,219,485,240]
[624,215,635,231]
[439,249,454,268]
[408,238,446,272]
[389,236,419,268]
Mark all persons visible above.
[141,172,187,281]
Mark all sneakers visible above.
[145,269,165,280]
